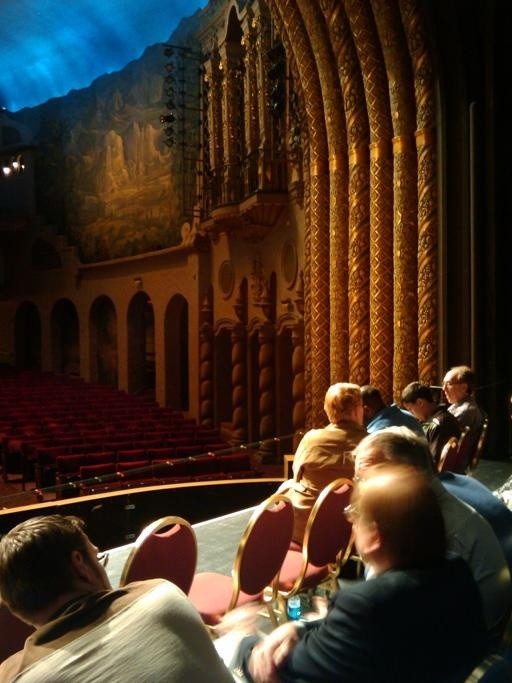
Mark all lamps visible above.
[3,153,21,174]
[159,42,207,220]
[259,15,301,164]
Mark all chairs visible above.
[119,516,197,596]
[269,478,353,598]
[189,495,295,626]
[289,536,354,566]
[438,419,489,474]
[466,653,508,683]
[1,367,286,512]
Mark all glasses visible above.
[94,552,110,567]
[343,506,362,524]
[440,381,453,385]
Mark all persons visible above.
[400,378,463,462]
[358,385,424,444]
[351,419,511,655]
[258,381,370,538]
[0,512,237,682]
[438,363,483,456]
[231,458,489,681]
